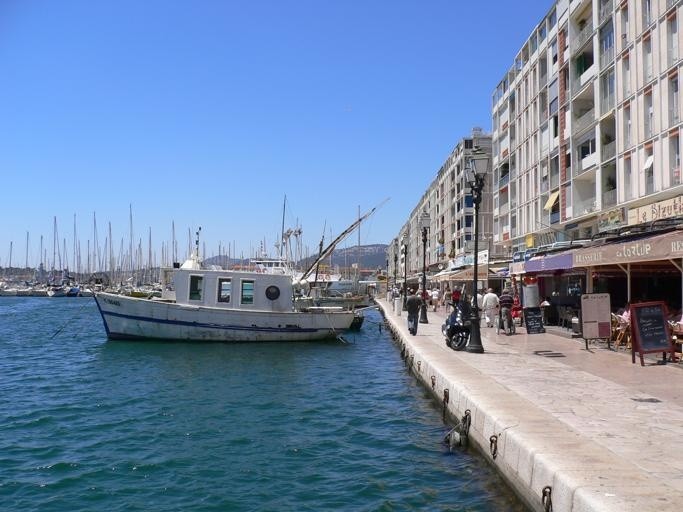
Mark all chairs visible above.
[557,305,577,331]
[611,313,631,352]
[668,320,683,364]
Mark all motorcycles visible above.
[442,283,482,350]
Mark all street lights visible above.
[402,233,409,311]
[394,244,399,285]
[419,210,431,322]
[463,145,489,352]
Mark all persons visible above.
[387,282,682,362]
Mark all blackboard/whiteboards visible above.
[524,307,545,334]
[630,301,674,354]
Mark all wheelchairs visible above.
[497,310,516,335]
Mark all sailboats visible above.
[1,202,162,298]
[166,195,369,307]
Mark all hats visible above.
[503,289,509,292]
[488,288,493,293]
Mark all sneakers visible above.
[410,328,416,335]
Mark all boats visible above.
[93,270,355,343]
